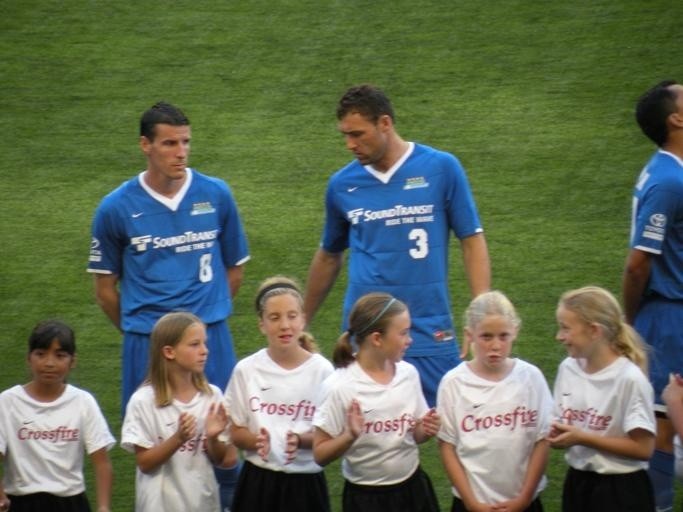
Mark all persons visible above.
[544,285,658,512]
[120,310,229,512]
[661,371,683,443]
[621,78,683,511]
[85,100,253,511]
[222,274,335,511]
[0,319,118,511]
[435,289,555,512]
[300,84,492,408]
[310,291,442,511]
